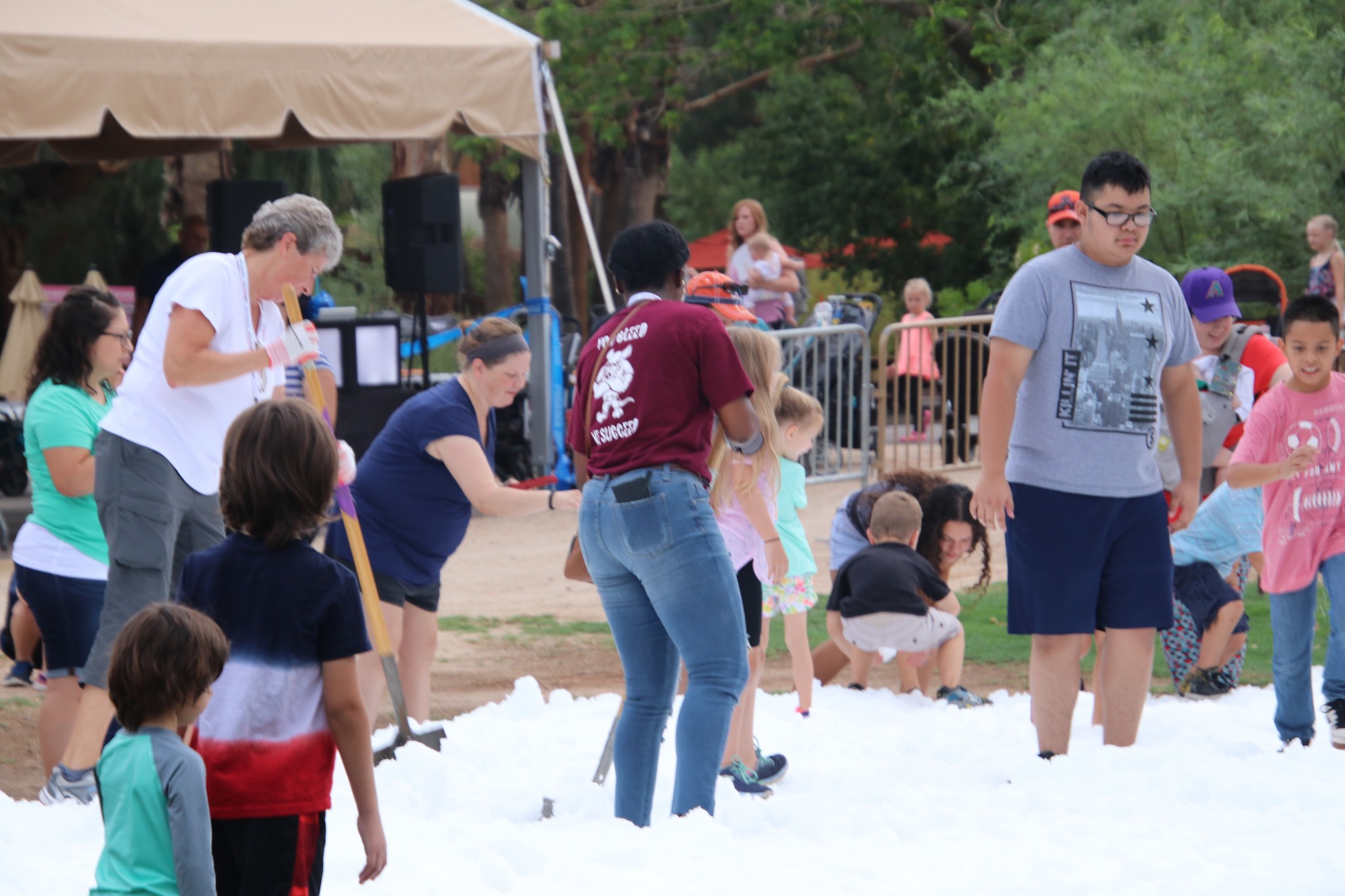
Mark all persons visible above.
[1044,190,1083,248]
[12,283,136,796]
[968,149,1204,763]
[0,569,47,691]
[37,195,343,809]
[1150,216,1345,753]
[177,399,388,896]
[87,599,230,896]
[328,316,583,735]
[567,219,758,835]
[685,199,994,793]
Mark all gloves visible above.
[333,439,357,488]
[262,319,320,370]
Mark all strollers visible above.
[780,290,883,476]
[931,290,1004,464]
[1223,265,1288,338]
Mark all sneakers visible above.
[38,764,98,807]
[937,685,993,709]
[1177,665,1238,700]
[1318,697,1345,751]
[718,735,788,799]
[1,659,35,687]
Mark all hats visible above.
[684,269,760,325]
[1045,190,1086,227]
[1177,267,1242,324]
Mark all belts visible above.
[591,463,703,480]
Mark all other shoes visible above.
[923,409,932,429]
[32,671,47,690]
[901,431,927,442]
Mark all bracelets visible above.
[547,490,557,510]
[764,537,779,543]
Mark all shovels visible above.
[281,280,449,769]
[540,416,727,817]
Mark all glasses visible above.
[690,281,748,299]
[1079,198,1158,227]
[101,329,134,347]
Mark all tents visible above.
[0,0,621,483]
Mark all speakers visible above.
[204,178,285,255]
[380,172,475,292]
